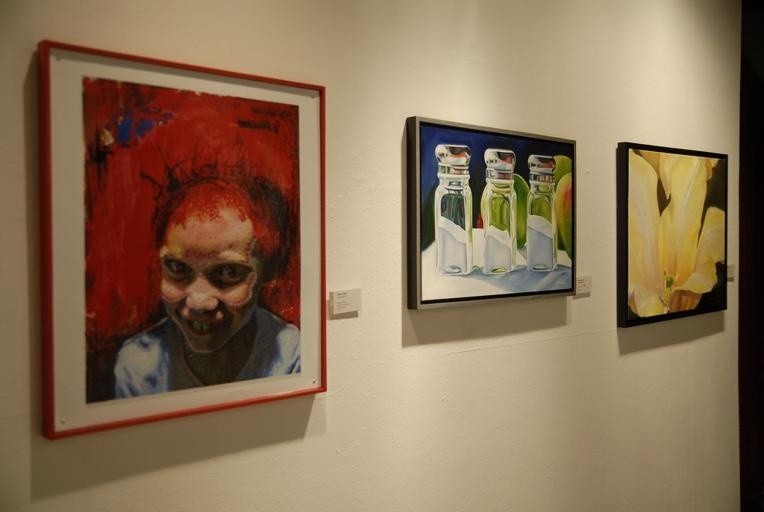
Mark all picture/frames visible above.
[36,39,327,441]
[407,116,576,311]
[617,142,728,328]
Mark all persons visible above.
[103,164,300,398]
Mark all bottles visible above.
[480,148,520,275]
[432,142,475,278]
[526,153,562,272]
